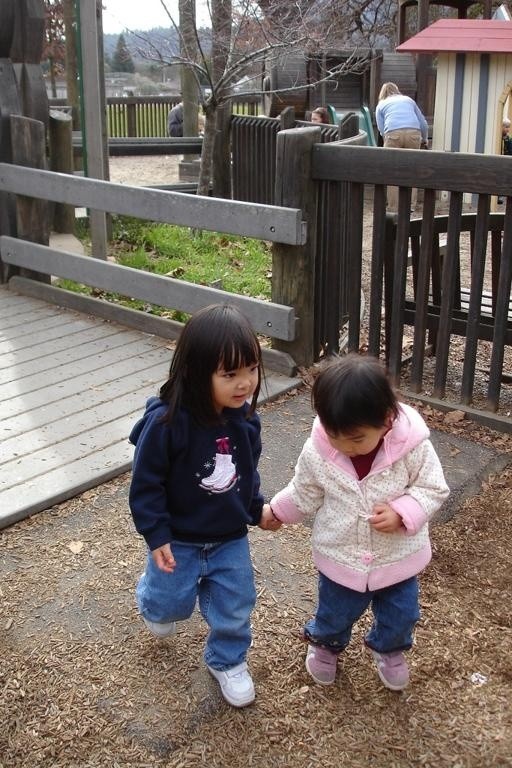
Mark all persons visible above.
[167,97,209,138]
[127,302,280,708]
[370,80,431,216]
[266,318,452,694]
[310,106,330,122]
[496,117,512,203]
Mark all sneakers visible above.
[371,646,410,692]
[306,643,338,686]
[207,661,255,707]
[141,569,175,637]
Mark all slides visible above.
[329,104,375,148]
